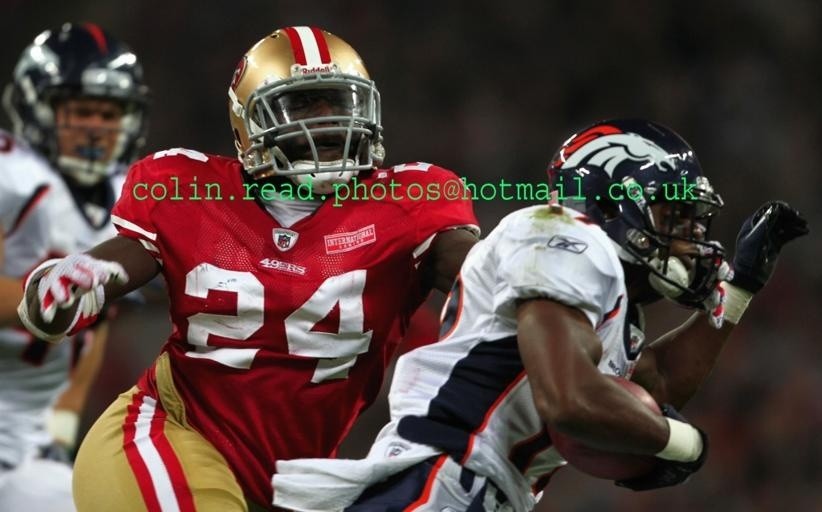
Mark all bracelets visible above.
[654,414,694,466]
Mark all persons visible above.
[0,20,150,512]
[17,26,485,512]
[270,116,810,512]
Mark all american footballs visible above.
[550,373,663,479]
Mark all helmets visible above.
[7,23,149,184]
[547,119,728,313]
[229,26,384,204]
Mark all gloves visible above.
[726,198,808,293]
[17,253,129,344]
[613,402,709,494]
